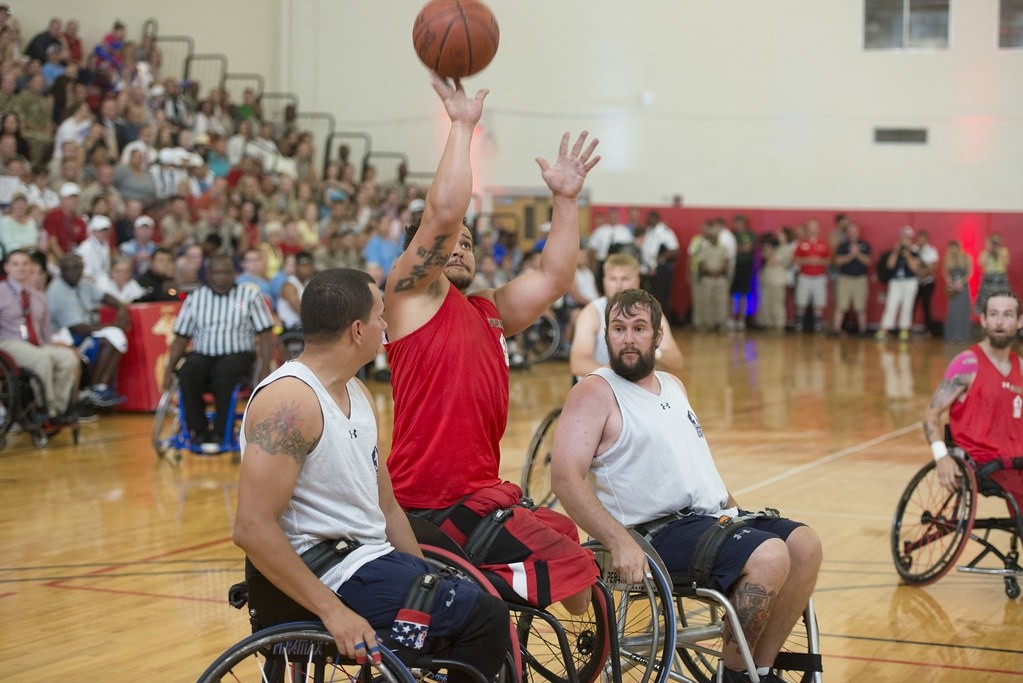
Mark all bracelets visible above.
[929,441,947,460]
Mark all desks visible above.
[102,300,250,411]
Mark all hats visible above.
[60,183,80,197]
[89,214,112,230]
[13,193,26,201]
[135,216,154,229]
[409,199,425,212]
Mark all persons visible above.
[232,267,511,683]
[381,69,600,616]
[549,290,822,683]
[0,0,1009,449]
[922,290,1023,520]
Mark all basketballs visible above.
[410,0,500,79]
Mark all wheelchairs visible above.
[195,496,622,683]
[520,375,582,508]
[153,351,266,464]
[891,423,1023,599]
[251,331,306,391]
[0,350,104,447]
[515,528,825,683]
[508,303,584,369]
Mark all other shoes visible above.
[900,332,908,339]
[875,330,886,338]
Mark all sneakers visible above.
[90,387,124,405]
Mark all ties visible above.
[21,290,39,345]
